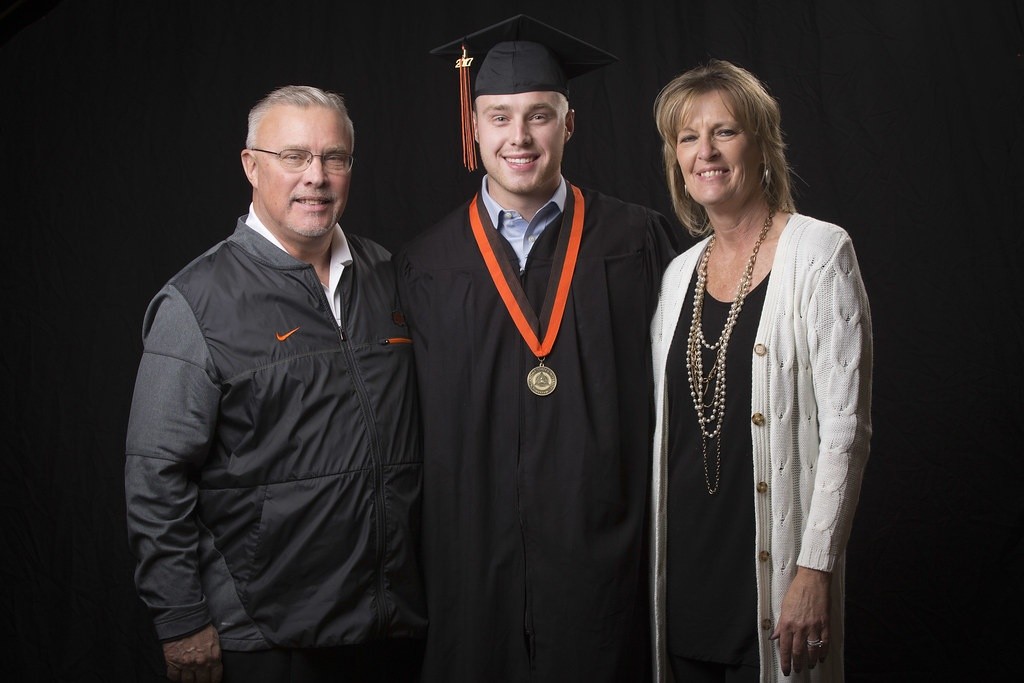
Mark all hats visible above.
[428,14,620,173]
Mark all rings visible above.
[807,639,822,647]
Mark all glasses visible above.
[249,148,354,175]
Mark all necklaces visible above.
[686,210,774,495]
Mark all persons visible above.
[648,59,874,683]
[124,85,430,683]
[392,16,683,683]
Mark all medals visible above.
[527,365,557,396]
